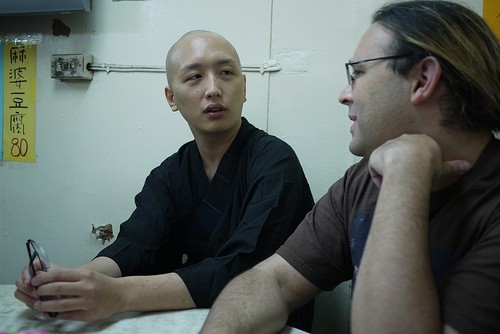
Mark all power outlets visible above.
[51,53,96,83]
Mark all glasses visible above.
[26,238,59,318]
[345,52,412,85]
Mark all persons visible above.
[198,0,500,334]
[14,30,316,334]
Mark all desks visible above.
[0,283,313,334]
